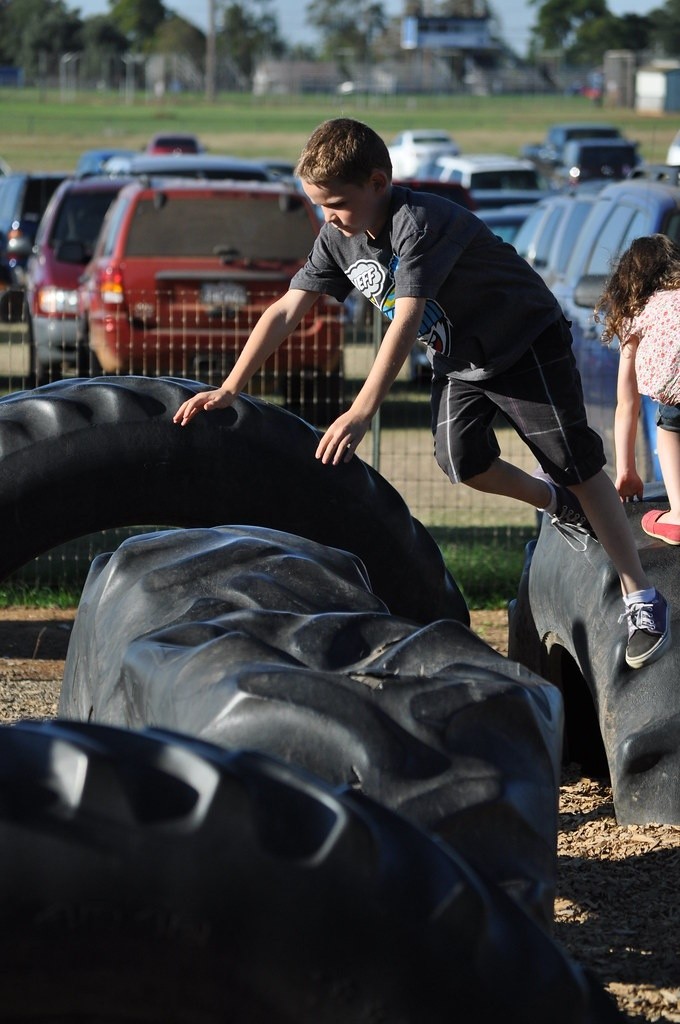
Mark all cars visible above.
[22,176,135,388]
[141,135,205,155]
[428,153,542,190]
[564,82,603,101]
[77,148,132,176]
[666,132,680,165]
[98,154,284,181]
[549,137,643,183]
[395,132,458,159]
[407,206,531,393]
[0,176,66,320]
[524,125,624,169]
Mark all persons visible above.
[172,117,680,671]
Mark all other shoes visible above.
[641,510,680,545]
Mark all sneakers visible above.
[618,585,671,669]
[532,470,600,551]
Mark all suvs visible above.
[75,172,349,416]
[512,182,611,300]
[562,162,680,484]
